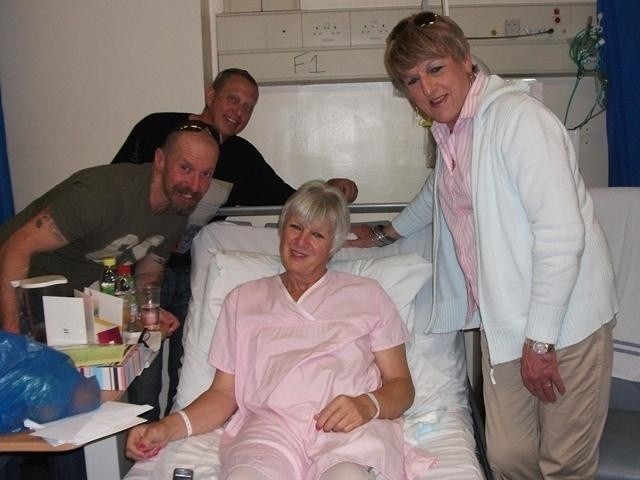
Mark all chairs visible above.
[584,186,640,480]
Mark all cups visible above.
[138,285,162,329]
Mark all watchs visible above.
[523,337,554,356]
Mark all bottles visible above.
[101,258,116,297]
[118,266,139,333]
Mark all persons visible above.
[125,180,418,480]
[1,121,224,479]
[107,68,358,419]
[336,9,620,480]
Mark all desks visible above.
[0,391,135,480]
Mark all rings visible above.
[543,383,553,392]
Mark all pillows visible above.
[198,246,433,360]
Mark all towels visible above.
[587,187,640,382]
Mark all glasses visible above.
[138,327,151,348]
[391,13,438,36]
[174,119,224,147]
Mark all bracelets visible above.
[364,390,380,422]
[366,224,395,247]
[176,411,192,438]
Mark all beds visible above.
[122,203,493,480]
